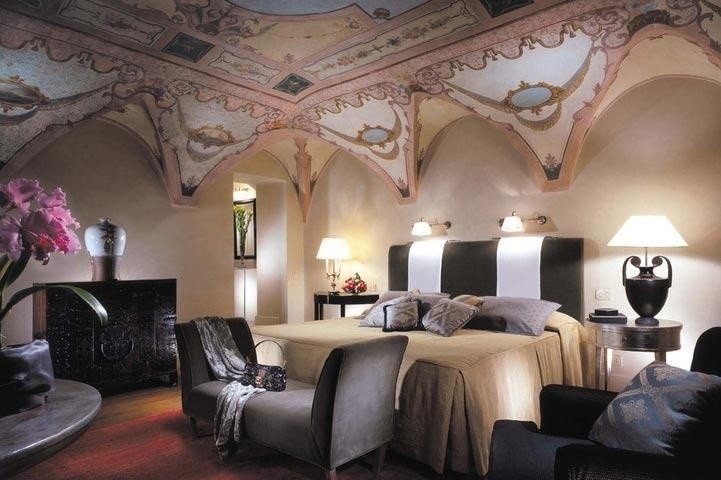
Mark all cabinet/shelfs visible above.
[34,279,178,389]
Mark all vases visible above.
[84,216,127,282]
[0,337,56,411]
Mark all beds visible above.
[250,238,595,477]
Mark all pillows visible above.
[354,289,562,338]
[586,361,720,458]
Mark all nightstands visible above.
[587,318,683,392]
[314,293,379,322]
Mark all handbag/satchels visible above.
[241,361,288,391]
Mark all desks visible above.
[1,378,103,479]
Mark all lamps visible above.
[316,235,351,295]
[608,215,689,326]
[498,209,546,233]
[410,217,451,236]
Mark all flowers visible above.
[0,176,109,347]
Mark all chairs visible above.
[491,325,720,480]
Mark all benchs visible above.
[173,317,409,480]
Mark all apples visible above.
[342,273,366,293]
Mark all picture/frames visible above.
[232,199,256,260]
